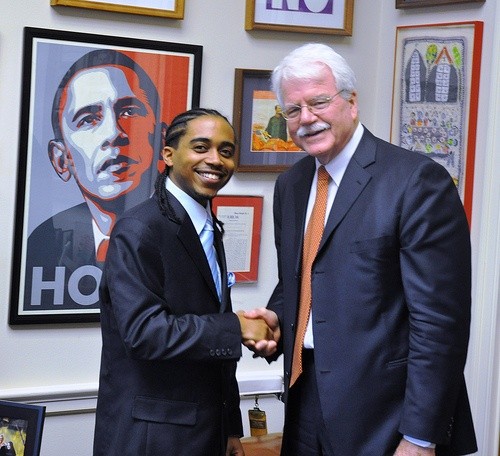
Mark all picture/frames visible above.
[9,27,203,325]
[245,0,353,36]
[50,0,185,19]
[390,21,484,232]
[233,68,309,172]
[0,400,46,456]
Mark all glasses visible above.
[282,90,342,119]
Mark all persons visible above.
[0,436,16,456]
[243,41,478,456]
[92,107,275,456]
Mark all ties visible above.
[200,217,223,305]
[288,165,330,389]
